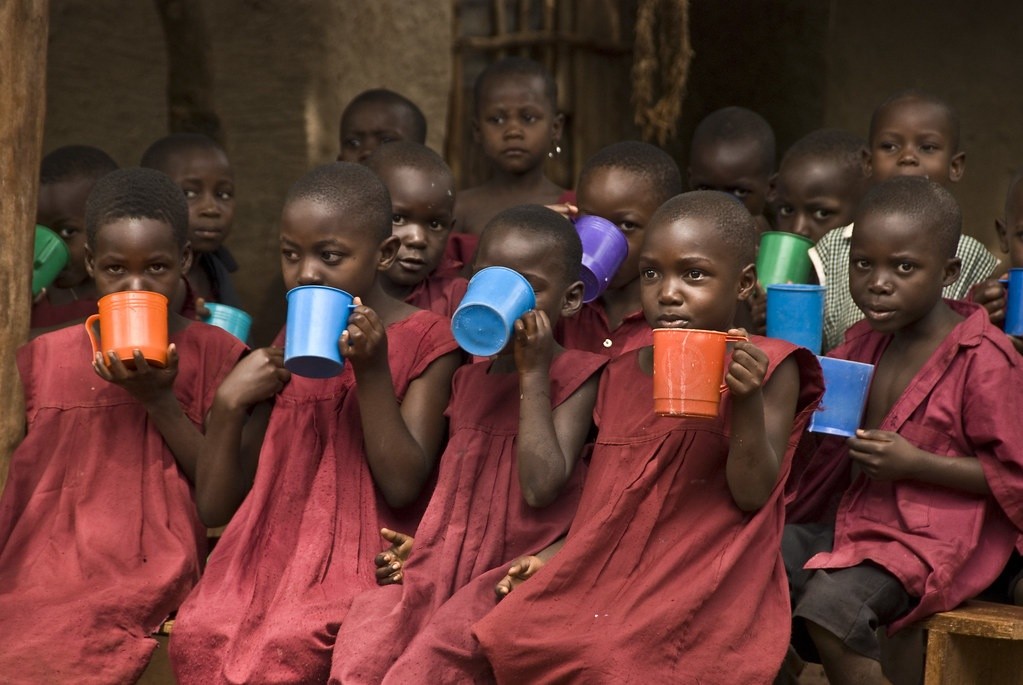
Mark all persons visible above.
[169,160,460,685]
[773,173,1023,685]
[0,165,252,685]
[30,57,1023,360]
[470,190,826,685]
[325,202,614,685]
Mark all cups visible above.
[32,223,71,299]
[766,284,828,357]
[753,231,816,294]
[651,327,748,422]
[200,302,254,346]
[568,215,628,303]
[283,285,359,379]
[807,356,876,439]
[450,266,535,356]
[84,290,168,370]
[998,267,1023,339]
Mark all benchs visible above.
[909,601,1023,685]
